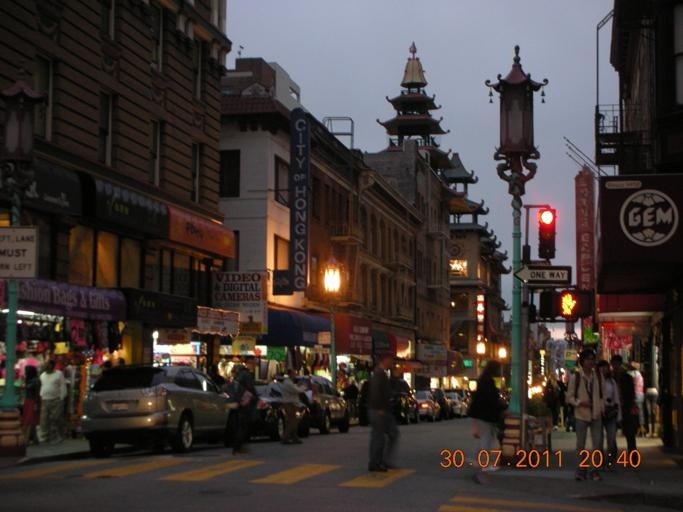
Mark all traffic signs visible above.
[513,265,570,289]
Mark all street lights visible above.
[321,259,340,394]
[474,342,506,387]
[486,46,548,462]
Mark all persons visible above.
[20,366,42,445]
[344,350,413,471]
[466,360,509,471]
[227,354,310,454]
[543,350,660,481]
[37,360,68,442]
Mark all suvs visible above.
[358,380,418,424]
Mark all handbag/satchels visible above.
[602,405,618,425]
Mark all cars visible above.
[415,388,510,422]
[79,365,352,454]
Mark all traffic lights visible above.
[538,290,593,322]
[537,209,556,262]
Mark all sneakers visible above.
[574,470,602,481]
[368,462,386,471]
